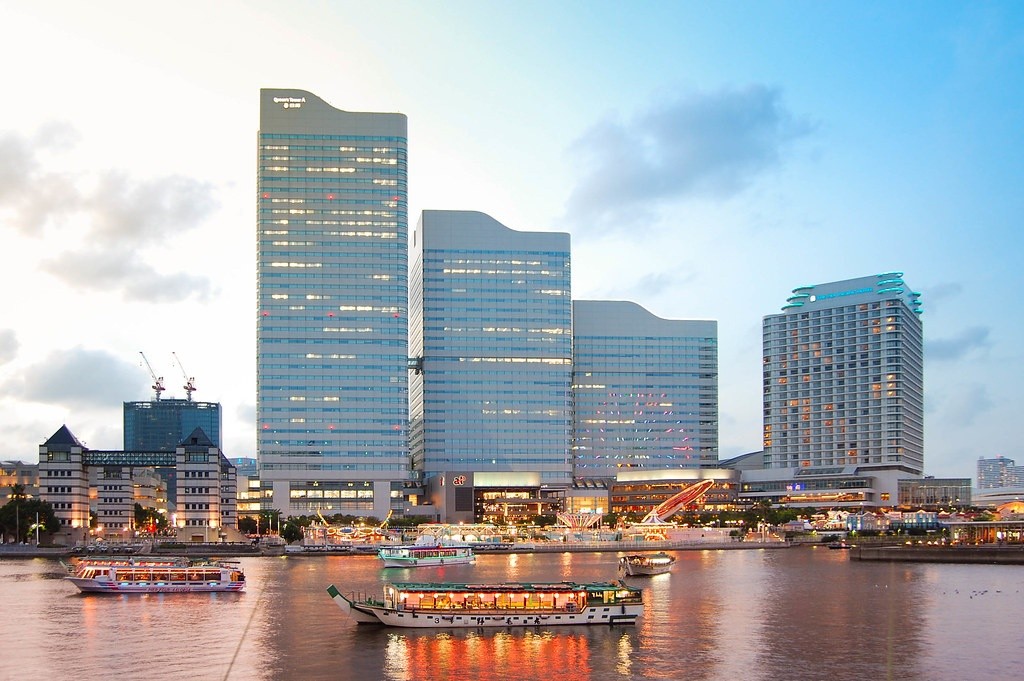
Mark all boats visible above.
[327,581,646,628]
[58,519,241,576]
[377,533,476,569]
[62,564,247,593]
[829,546,852,549]
[618,551,676,576]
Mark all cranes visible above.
[172,352,196,402]
[139,352,166,403]
